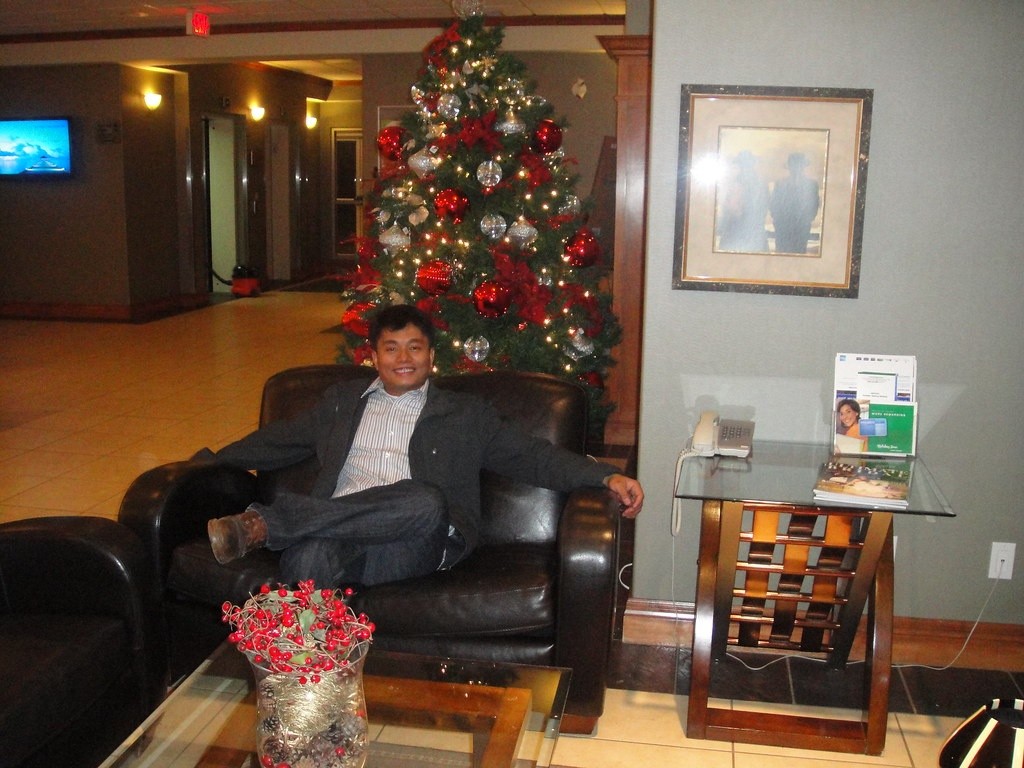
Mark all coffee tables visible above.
[95,633,576,768]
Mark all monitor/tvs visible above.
[0,115,73,179]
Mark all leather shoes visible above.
[208,512,268,564]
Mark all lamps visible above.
[938,697,1024,768]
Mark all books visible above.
[813,455,916,510]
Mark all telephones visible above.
[690,410,756,458]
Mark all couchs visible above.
[0,515,168,768]
[118,363,630,735]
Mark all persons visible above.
[836,399,868,452]
[188,304,644,590]
[714,152,822,253]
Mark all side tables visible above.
[674,438,959,757]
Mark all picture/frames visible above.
[669,82,876,301]
